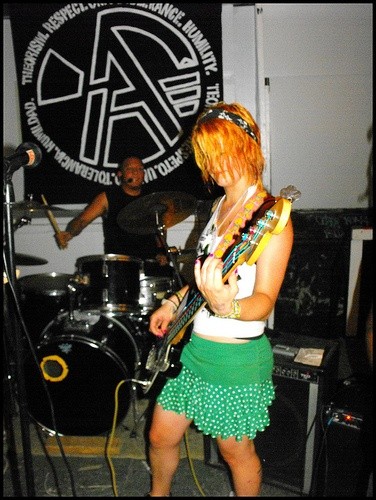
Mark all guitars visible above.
[138,183,301,398]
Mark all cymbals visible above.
[2,198,78,219]
[114,189,201,234]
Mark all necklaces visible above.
[207,187,251,236]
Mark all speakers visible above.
[208,334,339,495]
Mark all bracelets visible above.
[161,291,184,311]
[215,299,241,320]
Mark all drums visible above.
[6,273,72,305]
[20,311,136,438]
[73,253,138,302]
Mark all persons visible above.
[51,157,175,267]
[147,102,294,496]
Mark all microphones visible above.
[3,142,42,176]
[122,178,133,184]
